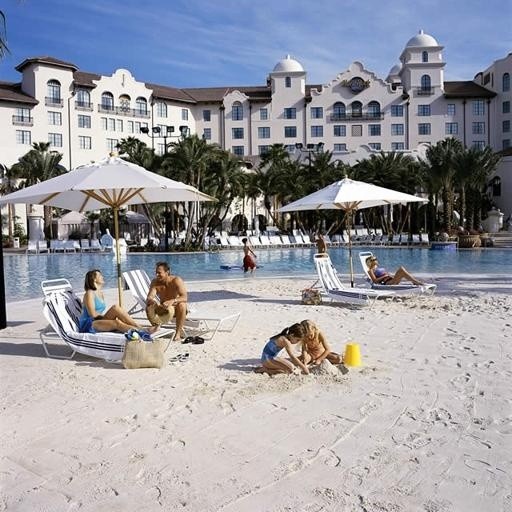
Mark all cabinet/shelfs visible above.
[122,270,240,341]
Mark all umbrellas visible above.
[0,152,222,312]
[272,174,430,282]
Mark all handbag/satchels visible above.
[122,335,165,369]
[301,289,322,305]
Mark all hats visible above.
[147,305,175,325]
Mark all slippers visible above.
[183,336,204,344]
[171,353,188,362]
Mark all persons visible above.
[254,322,310,376]
[241,238,258,273]
[310,232,327,270]
[145,261,191,341]
[364,255,425,287]
[78,269,162,334]
[298,319,343,368]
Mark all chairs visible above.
[301,254,420,312]
[40,279,177,361]
[26,228,429,255]
[359,252,437,308]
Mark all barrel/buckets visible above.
[345,343,361,367]
